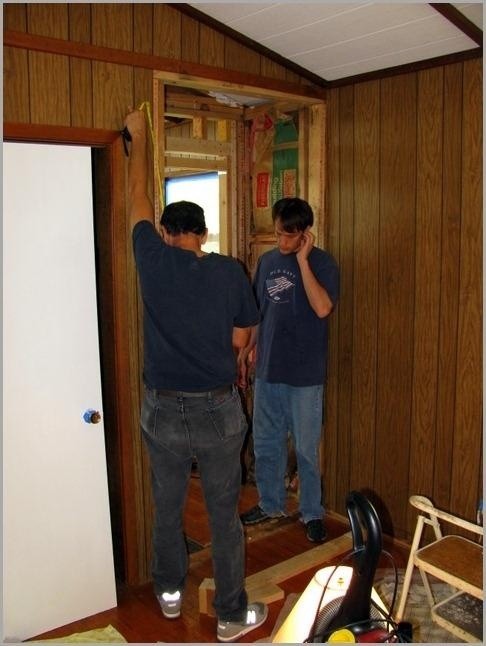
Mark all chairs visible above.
[392,494,485,643]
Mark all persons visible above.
[235,196,340,544]
[124,108,270,643]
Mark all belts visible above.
[143,382,233,399]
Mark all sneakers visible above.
[215,601,269,643]
[152,578,184,619]
[298,511,328,543]
[239,505,269,525]
